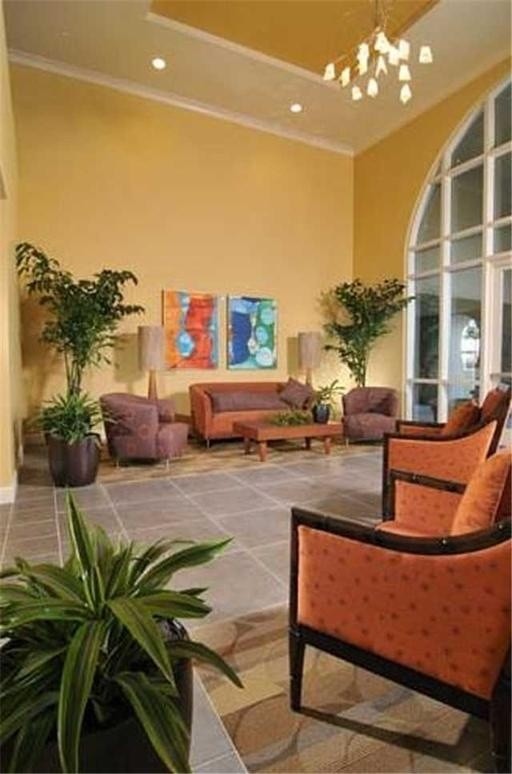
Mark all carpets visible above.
[95,436,384,488]
[183,598,511,771]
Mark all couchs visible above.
[188,381,313,449]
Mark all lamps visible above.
[137,325,163,399]
[298,330,320,386]
[319,0,436,107]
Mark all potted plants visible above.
[0,483,246,773]
[311,379,346,423]
[24,389,122,486]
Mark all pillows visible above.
[449,445,511,537]
[439,402,478,440]
[278,376,311,406]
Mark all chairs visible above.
[380,379,512,521]
[342,385,400,447]
[99,391,194,470]
[289,468,511,773]
